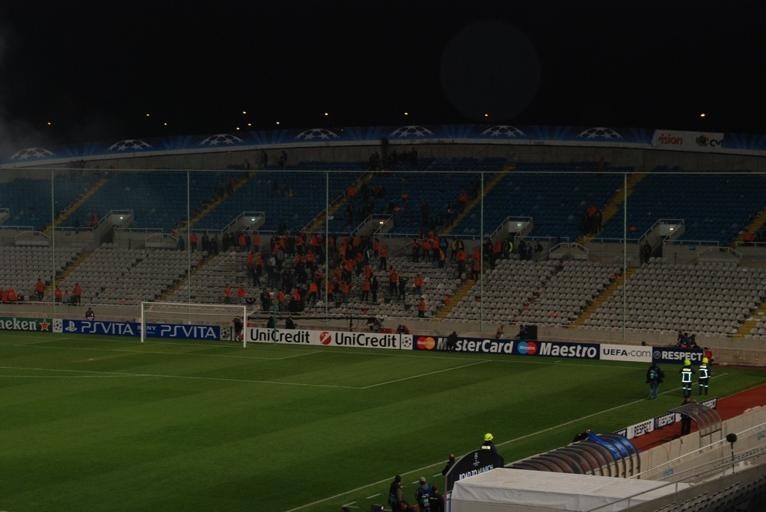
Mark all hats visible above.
[683,358,692,367]
[483,433,494,442]
[702,357,709,364]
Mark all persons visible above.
[573,432,584,441]
[388,475,403,512]
[680,395,692,436]
[442,453,463,491]
[679,360,696,398]
[428,487,444,512]
[415,477,432,512]
[646,363,664,400]
[581,427,592,440]
[399,501,416,512]
[480,433,496,454]
[699,357,712,396]
[0,149,754,370]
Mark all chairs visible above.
[617,463,766,512]
